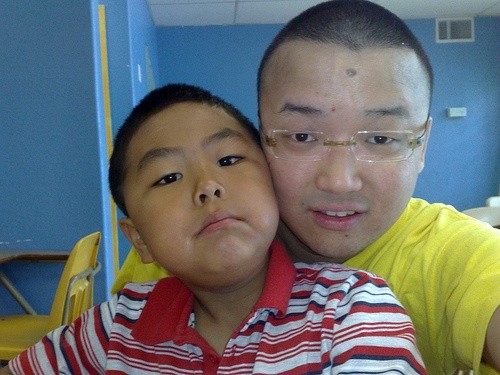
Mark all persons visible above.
[0,83,429,375]
[110,1,500,375]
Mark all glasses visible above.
[258,109,430,162]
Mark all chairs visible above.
[0,231,102,360]
